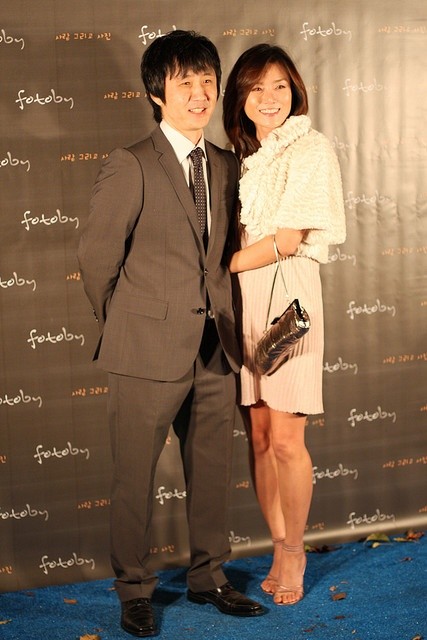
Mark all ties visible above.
[186,147,207,255]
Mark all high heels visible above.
[261,537,286,595]
[273,542,306,605]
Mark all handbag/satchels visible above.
[253,235,311,376]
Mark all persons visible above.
[223,44,344,605]
[77,30,263,636]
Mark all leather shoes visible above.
[120,597,158,637]
[186,582,270,617]
